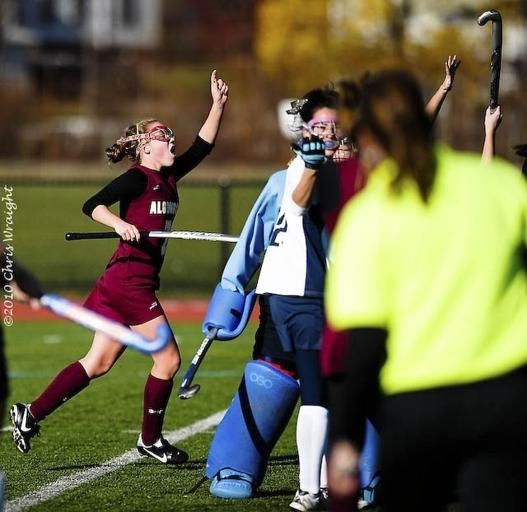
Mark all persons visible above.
[0,248,43,442]
[8,69,230,464]
[202,55,527,512]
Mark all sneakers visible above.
[289,486,368,512]
[209,469,253,499]
[10,403,37,453]
[137,432,188,464]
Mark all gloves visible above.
[290,135,325,172]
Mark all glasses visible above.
[304,117,338,137]
[125,127,173,142]
